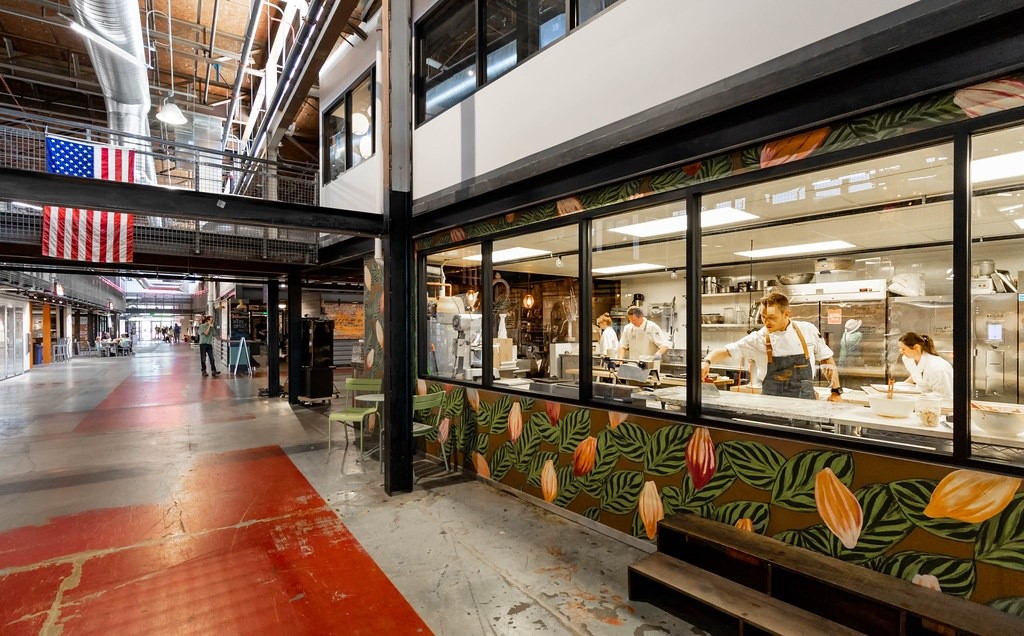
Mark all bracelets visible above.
[702,360,711,364]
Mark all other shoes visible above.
[202,370,209,376]
[212,370,221,376]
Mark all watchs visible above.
[830,387,843,394]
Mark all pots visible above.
[737,282,752,292]
[705,276,720,294]
[973,259,995,278]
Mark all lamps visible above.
[523,273,535,309]
[155,0,188,125]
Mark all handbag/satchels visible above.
[845,352,864,367]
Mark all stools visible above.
[53,337,71,362]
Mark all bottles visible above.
[916,398,941,427]
[724,306,746,324]
[498,314,508,339]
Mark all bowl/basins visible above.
[701,313,719,324]
[814,257,856,283]
[710,316,724,324]
[754,280,775,291]
[866,394,917,417]
[719,286,734,293]
[972,409,1024,438]
[775,272,816,285]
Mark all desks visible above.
[101,342,117,357]
[630,385,1024,453]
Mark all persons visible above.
[596,312,618,383]
[155,325,160,339]
[221,171,235,195]
[95,333,118,357]
[174,323,181,343]
[838,318,862,368]
[898,332,953,399]
[169,326,173,343]
[125,334,134,354]
[165,325,169,342]
[198,316,230,376]
[161,325,166,342]
[701,293,844,403]
[617,306,673,373]
[116,334,129,356]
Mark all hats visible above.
[845,319,862,334]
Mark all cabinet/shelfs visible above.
[699,291,766,331]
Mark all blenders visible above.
[633,293,643,307]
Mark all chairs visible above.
[97,338,132,358]
[379,390,450,485]
[329,378,382,463]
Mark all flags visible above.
[36,204,134,265]
[44,135,138,185]
